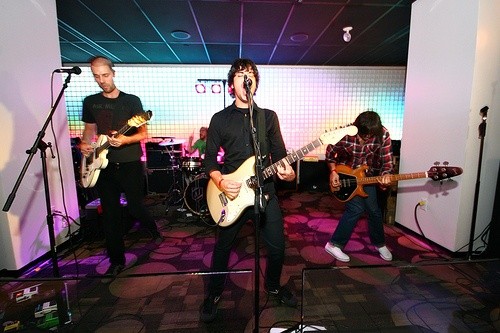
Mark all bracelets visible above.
[219,179,224,187]
[331,169,337,173]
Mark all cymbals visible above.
[158,138,185,146]
[151,136,175,139]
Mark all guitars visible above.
[80,109,153,190]
[329,161,463,204]
[205,124,359,228]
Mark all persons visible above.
[188,128,207,158]
[200,59,297,326]
[82,57,148,282]
[325,111,395,261]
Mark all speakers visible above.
[147,141,183,197]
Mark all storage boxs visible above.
[85,198,126,239]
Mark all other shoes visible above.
[150,231,162,245]
[111,262,128,273]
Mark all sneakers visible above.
[325,242,350,262]
[374,245,392,261]
[264,286,296,307]
[203,288,224,313]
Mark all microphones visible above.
[480,106,489,114]
[56,65,81,75]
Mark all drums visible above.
[179,156,202,171]
[183,173,211,217]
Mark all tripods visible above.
[162,145,184,217]
